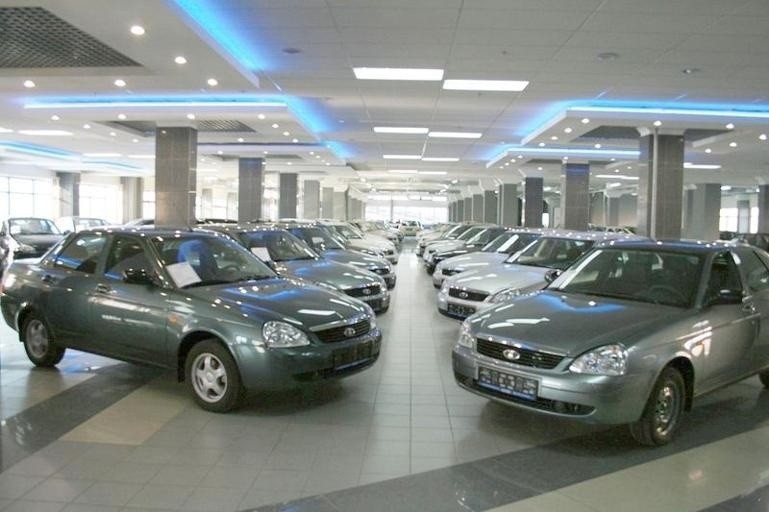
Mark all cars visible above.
[316,219,399,265]
[195,220,390,315]
[415,223,461,256]
[433,227,546,289]
[438,229,625,321]
[398,218,423,236]
[381,220,405,240]
[452,237,769,446]
[277,218,396,290]
[428,226,510,276]
[0,217,70,279]
[52,216,111,241]
[349,221,400,250]
[0,228,382,411]
